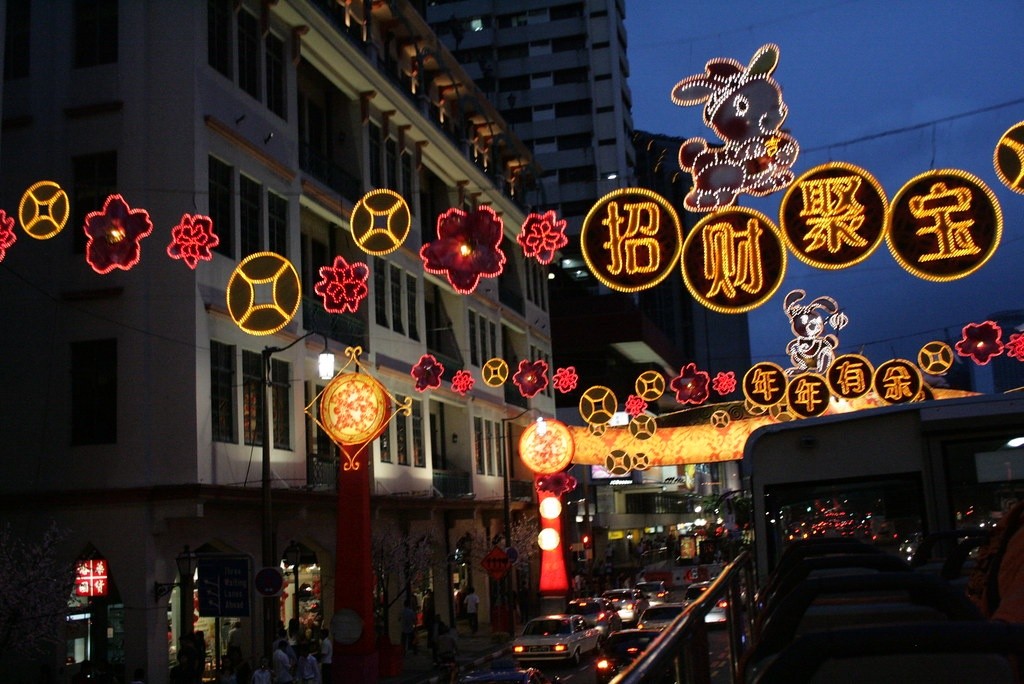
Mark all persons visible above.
[398,588,480,684]
[167,615,335,684]
[517,532,677,625]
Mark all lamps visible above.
[153,544,197,604]
[282,540,301,577]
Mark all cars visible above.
[512,614,604,666]
[564,598,624,643]
[637,602,690,630]
[594,627,677,684]
[682,575,747,628]
[601,588,651,628]
[636,580,672,606]
[465,667,561,684]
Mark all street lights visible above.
[258,329,336,669]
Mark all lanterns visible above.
[536,499,564,552]
[693,523,739,536]
